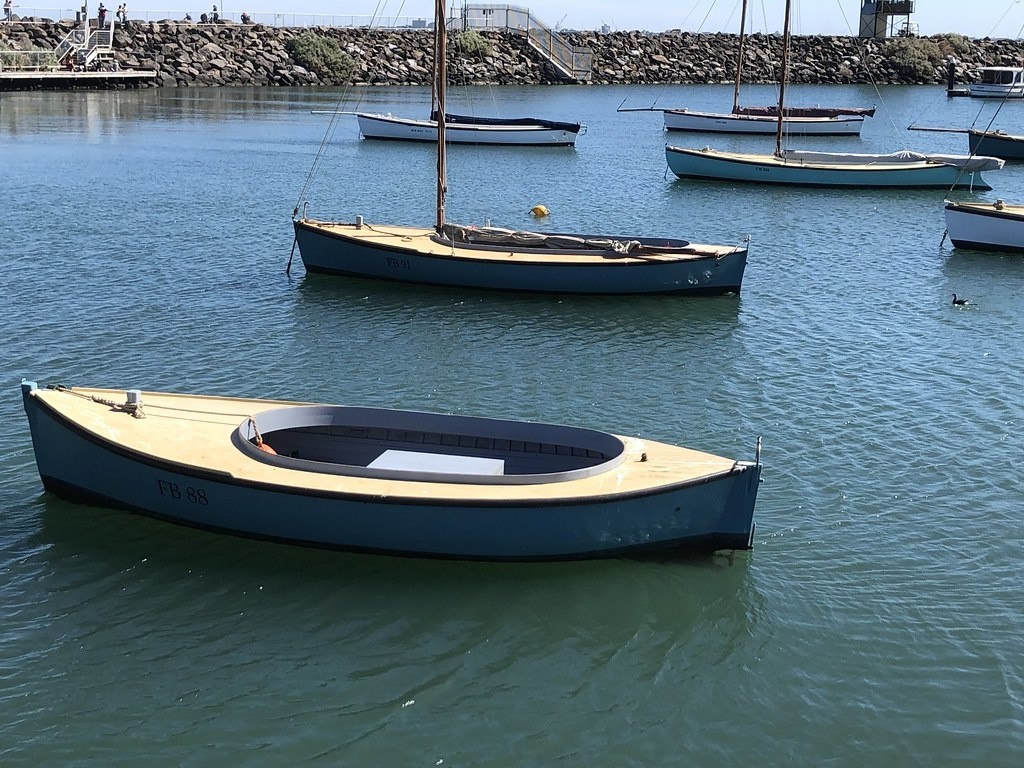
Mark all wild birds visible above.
[951,294,969,306]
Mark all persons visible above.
[117,5,124,24]
[122,3,128,23]
[98,2,109,30]
[200,13,208,22]
[8,0,13,21]
[213,5,218,22]
[241,12,250,23]
[184,12,192,21]
[3,0,11,21]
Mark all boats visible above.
[357,1,588,152]
[968,64,1024,99]
[17,374,769,565]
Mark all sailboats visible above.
[940,61,1023,256]
[906,1,1023,160]
[286,0,755,299]
[617,0,879,138]
[660,0,1005,193]
